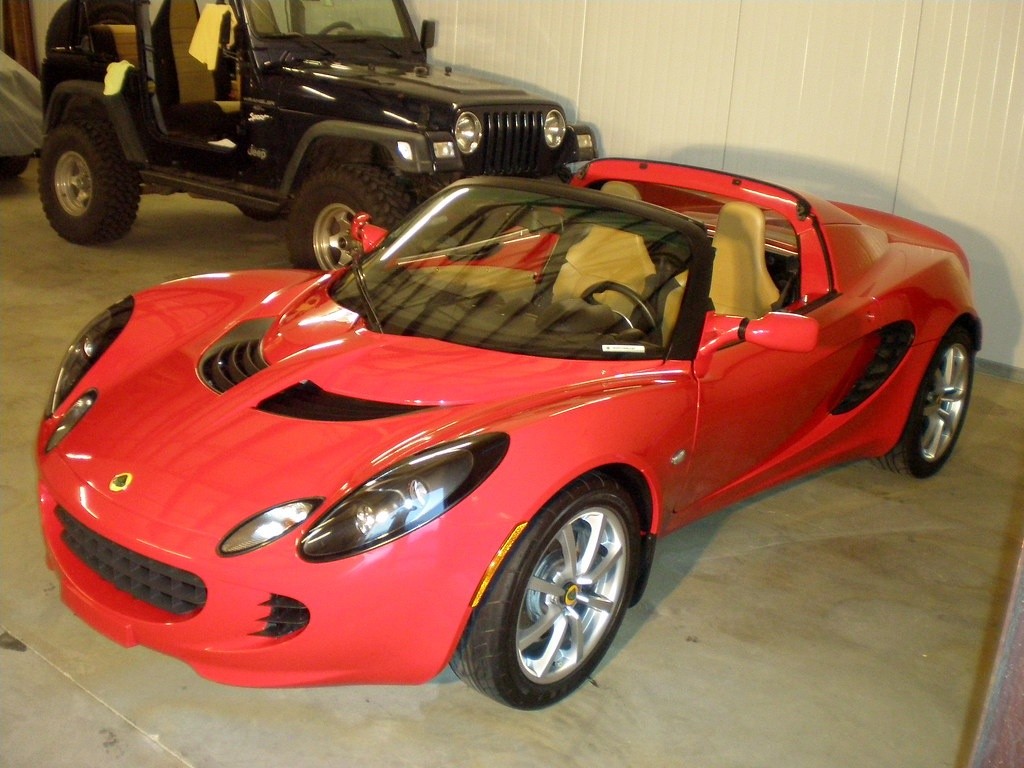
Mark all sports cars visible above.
[32,155,987,710]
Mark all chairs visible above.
[549,180,656,322]
[229,0,282,85]
[91,24,138,67]
[150,0,239,138]
[661,201,779,350]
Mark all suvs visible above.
[34,0,600,272]
